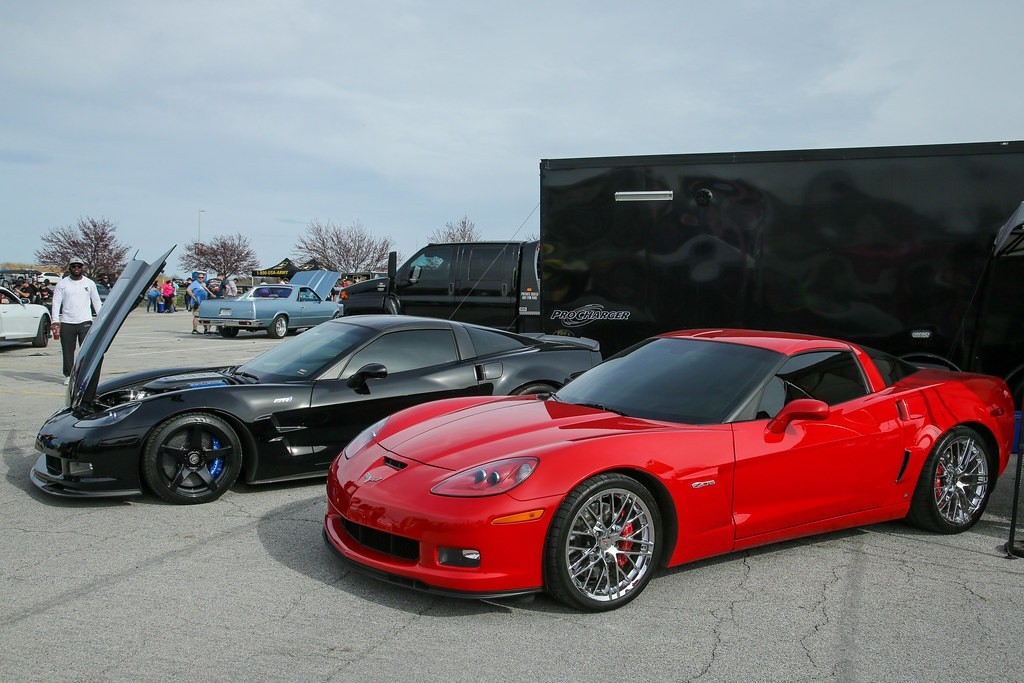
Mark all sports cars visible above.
[29,243,601,501]
[323,326,1016,609]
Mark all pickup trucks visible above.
[192,282,343,339]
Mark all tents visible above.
[251,258,299,286]
[297,258,338,272]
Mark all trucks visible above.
[341,271,388,287]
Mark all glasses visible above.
[198,277,204,279]
[71,263,82,268]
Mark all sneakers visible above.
[63,376,71,386]
[192,331,203,334]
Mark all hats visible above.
[69,258,84,264]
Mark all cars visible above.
[172,278,184,287]
[0,270,41,287]
[90,283,109,315]
[36,272,60,286]
[0,286,52,350]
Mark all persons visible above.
[102,275,112,290]
[206,273,230,300]
[184,277,193,310]
[226,278,239,298]
[51,256,104,386]
[259,277,267,285]
[277,276,289,285]
[186,273,212,335]
[13,278,53,302]
[146,277,180,314]
[331,278,349,301]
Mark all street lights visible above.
[198,209,207,241]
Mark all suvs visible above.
[332,241,543,336]
